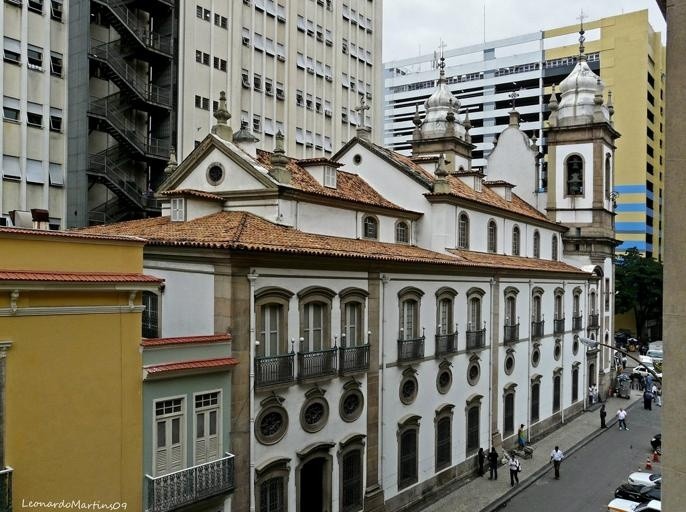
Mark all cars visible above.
[607,471,663,512]
[630,341,663,383]
[649,432,661,457]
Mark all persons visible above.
[487,447,498,480]
[616,408,627,430]
[509,455,519,487]
[628,372,661,410]
[600,404,608,428]
[651,434,661,451]
[551,446,563,479]
[518,424,525,448]
[589,383,597,404]
[479,448,485,476]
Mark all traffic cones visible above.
[635,450,660,472]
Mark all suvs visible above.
[613,347,627,369]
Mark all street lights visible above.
[578,336,663,389]
